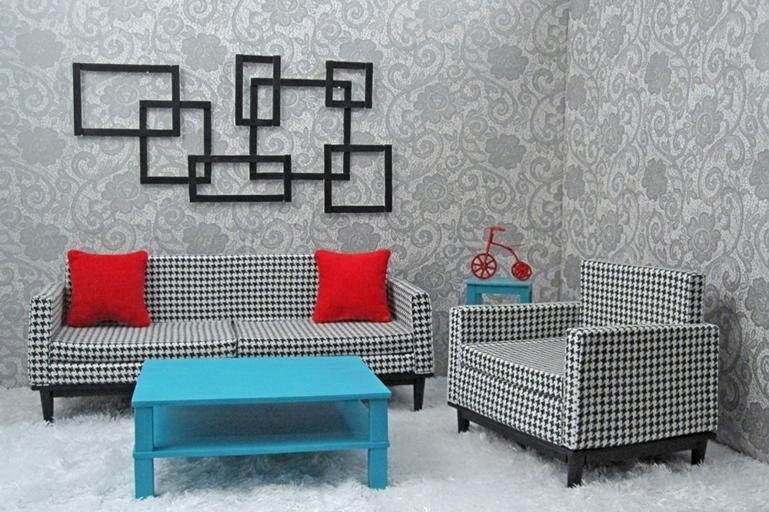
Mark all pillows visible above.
[312,251,393,324]
[65,251,151,328]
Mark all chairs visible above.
[445,259,720,489]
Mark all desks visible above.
[465,277,535,308]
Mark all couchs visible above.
[24,249,432,420]
